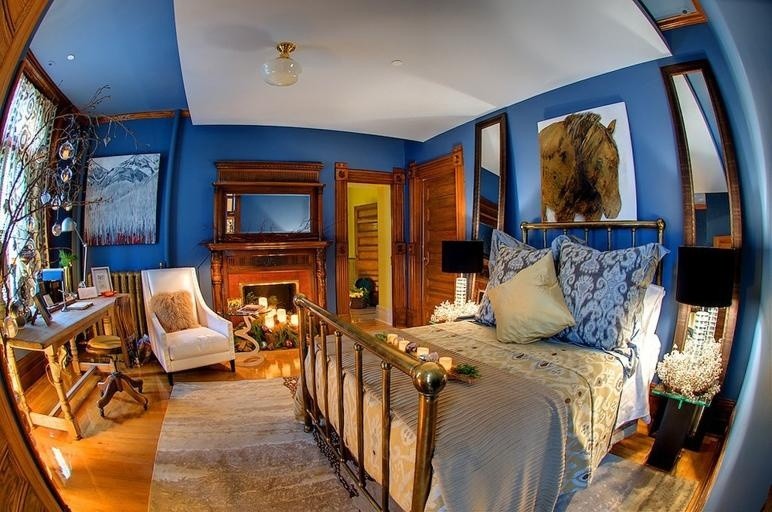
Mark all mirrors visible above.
[465,112,509,306]
[660,57,745,372]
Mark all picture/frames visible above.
[80,153,161,246]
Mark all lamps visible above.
[676,245,738,355]
[259,41,302,87]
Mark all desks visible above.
[5,295,131,441]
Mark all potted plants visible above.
[350,287,369,309]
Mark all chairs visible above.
[141,267,235,386]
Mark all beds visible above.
[294,219,672,511]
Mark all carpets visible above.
[149,376,699,512]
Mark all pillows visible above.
[150,290,201,333]
[474,228,666,350]
[441,239,483,306]
[144,287,199,331]
